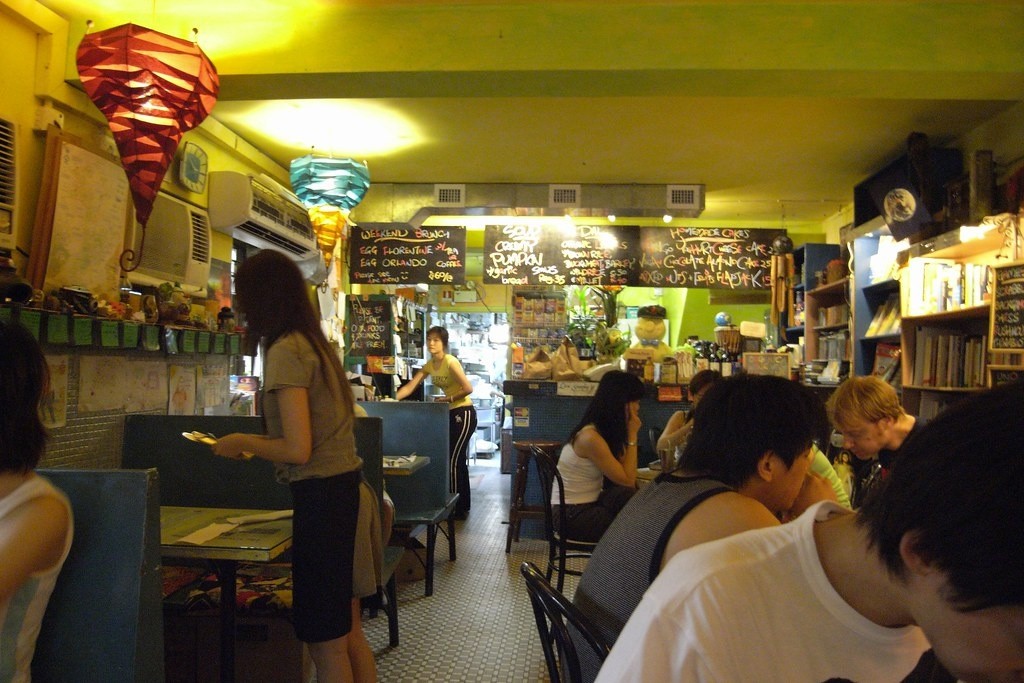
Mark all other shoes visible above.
[455,510,468,520]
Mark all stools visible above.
[506,441,563,554]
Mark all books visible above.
[871,344,902,392]
[906,254,989,314]
[912,325,987,387]
[863,298,902,338]
[816,303,849,327]
[817,332,849,359]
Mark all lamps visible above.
[453,280,477,303]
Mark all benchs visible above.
[33,401,461,683]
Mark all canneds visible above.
[790,363,805,384]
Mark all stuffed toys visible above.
[621,315,673,364]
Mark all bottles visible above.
[720,342,732,376]
[709,342,720,374]
[696,341,709,371]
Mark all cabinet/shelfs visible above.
[784,208,1024,424]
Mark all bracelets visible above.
[450,394,453,402]
[626,441,638,446]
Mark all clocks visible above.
[177,141,208,195]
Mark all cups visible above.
[660,449,675,473]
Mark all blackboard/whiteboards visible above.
[744,352,791,380]
[987,259,1024,354]
[483,225,639,285]
[640,228,788,289]
[348,226,466,283]
[348,301,391,357]
[33,133,138,312]
[987,363,1024,389]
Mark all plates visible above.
[818,380,840,384]
[818,377,840,380]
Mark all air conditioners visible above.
[208,171,316,262]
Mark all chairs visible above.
[530,443,605,642]
[517,561,612,683]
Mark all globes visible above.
[716,312,732,326]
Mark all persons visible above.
[589,371,1023,683]
[550,368,643,548]
[212,246,386,683]
[0,312,75,683]
[827,375,936,484]
[832,452,853,499]
[656,369,726,461]
[397,326,477,520]
[560,373,838,683]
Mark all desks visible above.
[158,505,295,683]
[381,454,431,476]
